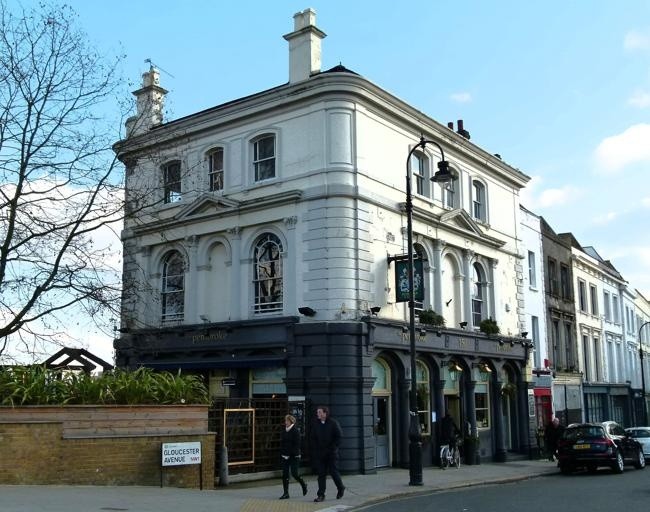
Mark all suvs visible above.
[556,420,647,473]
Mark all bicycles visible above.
[439,434,463,469]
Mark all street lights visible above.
[404,132,460,487]
[638,321,649,424]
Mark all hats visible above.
[285,414,296,424]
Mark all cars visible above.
[623,427,650,460]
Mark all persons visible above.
[550,417,565,460]
[441,411,462,460]
[311,407,341,499]
[279,414,308,499]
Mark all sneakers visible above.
[337,488,344,499]
[314,496,324,502]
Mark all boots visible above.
[297,478,307,496]
[279,479,290,499]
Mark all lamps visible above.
[460,322,467,329]
[471,362,493,382]
[371,306,380,315]
[441,360,463,381]
[298,306,316,317]
[199,315,211,323]
[117,328,131,333]
[522,332,528,339]
